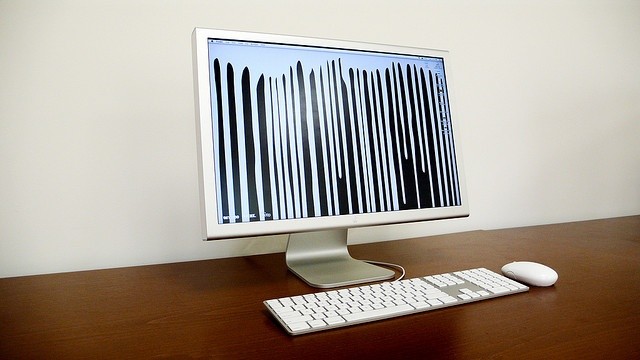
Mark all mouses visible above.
[501,257,560,291]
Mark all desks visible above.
[0,215,639,360]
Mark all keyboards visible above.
[260,266,530,338]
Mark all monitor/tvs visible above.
[191,26,469,287]
